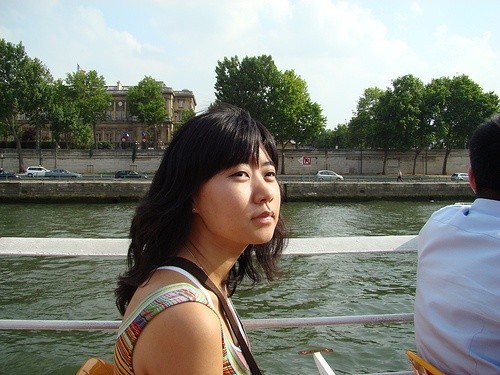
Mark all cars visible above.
[451,173,470,182]
[45,168,82,179]
[0,169,20,180]
[25,166,50,177]
[114,170,148,179]
[313,170,344,182]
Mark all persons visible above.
[397,169,403,182]
[113,102,290,375]
[413,112,500,375]
